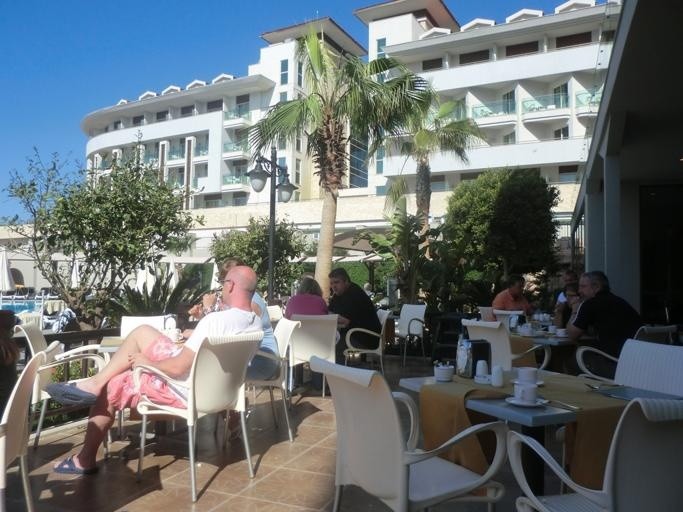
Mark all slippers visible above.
[45,382,97,405]
[53,454,99,474]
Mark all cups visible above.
[169,328,183,341]
[549,326,556,332]
[519,367,536,382]
[514,383,538,403]
[557,329,566,336]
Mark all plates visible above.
[506,397,549,406]
[512,378,544,385]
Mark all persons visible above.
[567,270,642,380]
[554,282,584,328]
[284,276,329,391]
[491,274,533,317]
[553,269,579,308]
[188,258,282,432]
[45,265,264,476]
[327,267,383,365]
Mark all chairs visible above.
[133,332,264,502]
[0,341,64,512]
[577,326,678,377]
[309,356,507,512]
[116,314,178,439]
[461,320,551,370]
[214,319,301,452]
[284,314,339,410]
[577,340,683,397]
[394,304,426,367]
[247,306,283,399]
[478,309,528,335]
[12,322,113,468]
[343,309,392,379]
[508,397,683,512]
[38,300,67,332]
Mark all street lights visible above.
[244,145,298,305]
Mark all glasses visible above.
[564,294,578,299]
[219,279,231,285]
